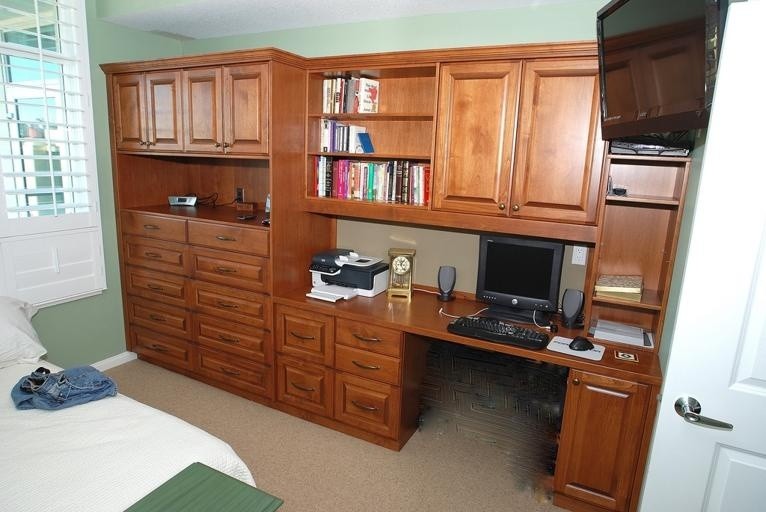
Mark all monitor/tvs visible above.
[476,235,565,324]
[594,0,731,147]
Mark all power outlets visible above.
[570,244,587,266]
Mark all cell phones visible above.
[237,213,257,220]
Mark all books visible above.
[595,272,646,292]
[313,156,432,208]
[596,291,642,302]
[321,76,377,154]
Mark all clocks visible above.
[386,248,416,300]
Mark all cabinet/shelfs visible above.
[304,47,437,228]
[112,68,186,159]
[186,61,307,162]
[582,144,694,353]
[430,41,605,246]
[116,201,339,403]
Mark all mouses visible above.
[568,335,595,351]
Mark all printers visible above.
[305,248,390,305]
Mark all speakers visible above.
[437,265,457,302]
[562,288,586,330]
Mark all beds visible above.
[1,288,257,510]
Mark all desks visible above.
[273,280,662,512]
[123,461,285,512]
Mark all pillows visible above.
[1,294,49,366]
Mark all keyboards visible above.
[446,316,550,352]
